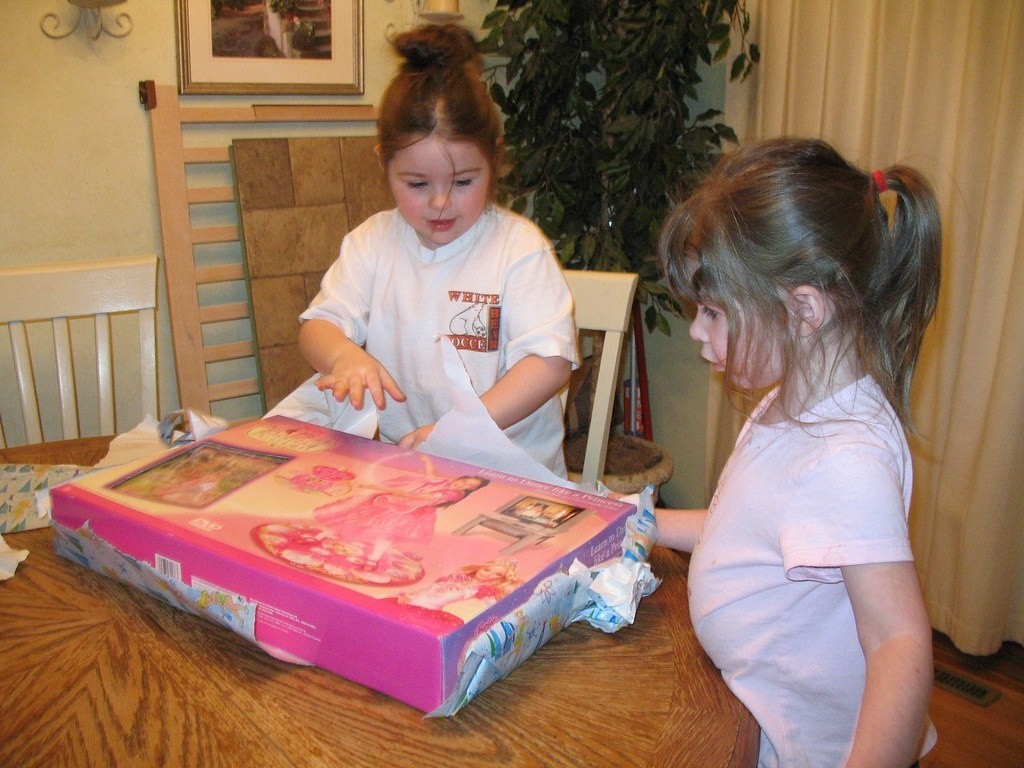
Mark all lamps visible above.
[382,0,465,48]
[39,1,133,40]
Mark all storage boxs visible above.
[48,414,638,720]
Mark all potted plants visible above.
[471,0,762,508]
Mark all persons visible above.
[654,136,941,768]
[298,25,581,481]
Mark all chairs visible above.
[0,254,163,446]
[551,269,640,491]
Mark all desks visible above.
[0,434,760,768]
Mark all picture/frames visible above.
[173,0,365,95]
